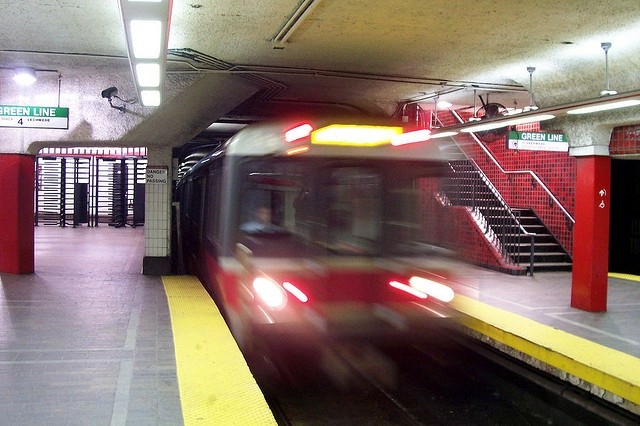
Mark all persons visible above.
[239,202,286,235]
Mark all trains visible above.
[175,116,476,426]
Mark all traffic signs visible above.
[506,131,569,152]
[0,106,68,128]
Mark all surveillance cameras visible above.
[101,86,119,99]
